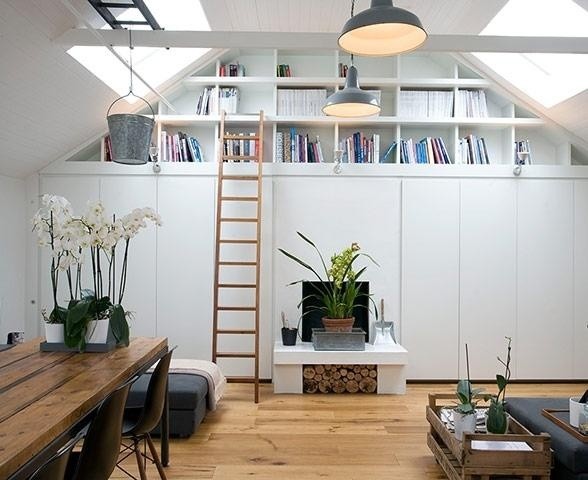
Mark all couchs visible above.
[0,336,227,440]
[500,395,588,480]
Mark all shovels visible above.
[371,299,398,347]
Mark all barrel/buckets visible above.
[103,90,159,166]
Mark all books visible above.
[469,440,534,452]
[105,135,159,163]
[162,61,533,165]
[435,405,491,433]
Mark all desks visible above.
[272,340,408,396]
[0,339,169,480]
[425,392,554,480]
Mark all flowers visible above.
[28,191,163,347]
[277,232,382,319]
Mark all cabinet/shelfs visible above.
[517,178,587,380]
[38,176,157,370]
[38,47,588,174]
[402,177,516,383]
[157,175,272,381]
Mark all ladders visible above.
[212,109,265,403]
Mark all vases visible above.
[569,397,588,428]
[322,316,356,333]
[44,316,114,344]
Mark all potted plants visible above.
[450,343,485,442]
[475,335,511,435]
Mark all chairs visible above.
[26,430,85,480]
[82,344,176,480]
[63,375,137,480]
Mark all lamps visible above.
[334,150,344,174]
[150,147,160,172]
[338,0,429,58]
[321,53,381,118]
[512,151,528,176]
